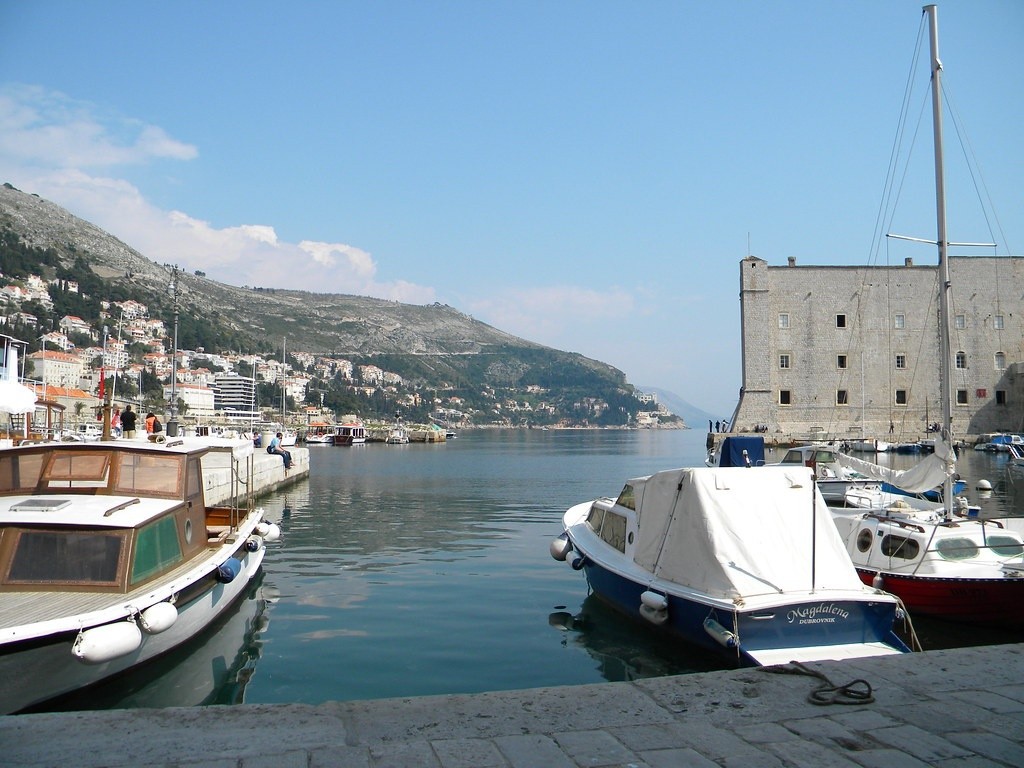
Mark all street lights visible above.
[166,264,181,436]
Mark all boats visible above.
[385,410,410,444]
[843,486,980,517]
[68,423,103,437]
[0,387,280,717]
[761,445,883,503]
[850,472,967,499]
[304,423,335,443]
[917,439,960,450]
[550,463,920,667]
[974,435,1024,452]
[446,430,456,439]
[897,443,921,452]
[334,425,367,445]
[705,437,765,467]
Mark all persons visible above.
[269,432,295,469]
[929,422,941,432]
[120,404,137,439]
[722,420,726,432]
[253,432,261,447]
[889,421,894,433]
[715,420,720,432]
[754,424,768,433]
[146,413,156,434]
[709,419,713,433]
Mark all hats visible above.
[276,433,283,437]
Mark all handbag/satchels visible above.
[153,417,162,432]
[267,445,275,454]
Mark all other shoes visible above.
[287,466,291,469]
[290,463,295,466]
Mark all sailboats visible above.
[236,336,299,448]
[817,5,1023,647]
[850,351,889,452]
[192,375,225,438]
